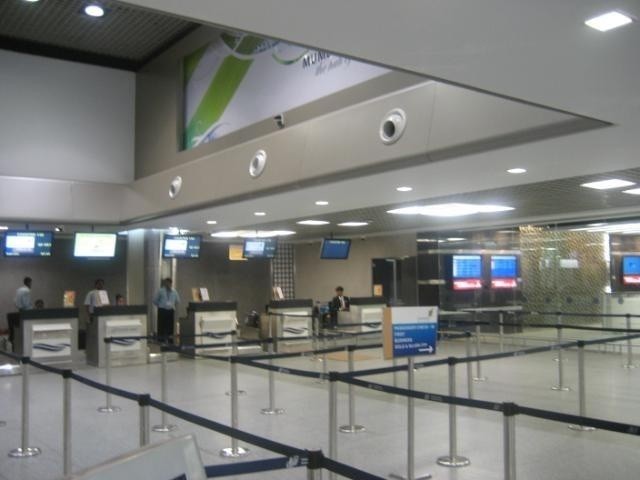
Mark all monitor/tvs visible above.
[321,239,351,259]
[162,236,202,258]
[4,230,54,258]
[242,239,278,258]
[73,231,118,261]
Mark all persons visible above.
[330,287,350,327]
[85,279,109,322]
[115,296,125,305]
[14,276,33,311]
[155,278,181,341]
[35,300,46,309]
